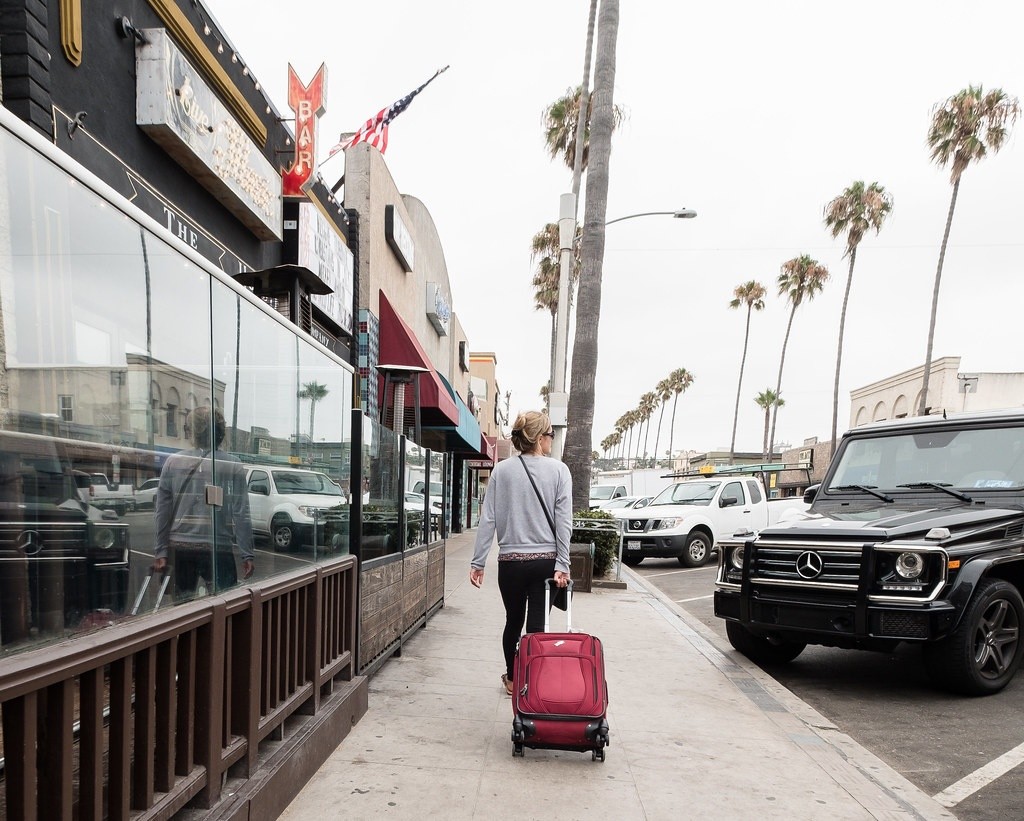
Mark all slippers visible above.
[501,674,513,695]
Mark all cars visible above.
[361,490,444,528]
[591,495,655,518]
[131,478,160,509]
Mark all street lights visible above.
[548,193,699,460]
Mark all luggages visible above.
[511,577,610,763]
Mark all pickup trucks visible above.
[614,461,816,568]
[238,463,348,553]
[73,470,133,515]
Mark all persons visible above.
[468,411,574,697]
[150,407,256,606]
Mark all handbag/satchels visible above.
[549,579,573,611]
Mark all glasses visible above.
[542,430,555,440]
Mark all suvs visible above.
[713,408,1023,695]
[0,428,131,645]
[413,480,444,522]
[589,484,627,512]
